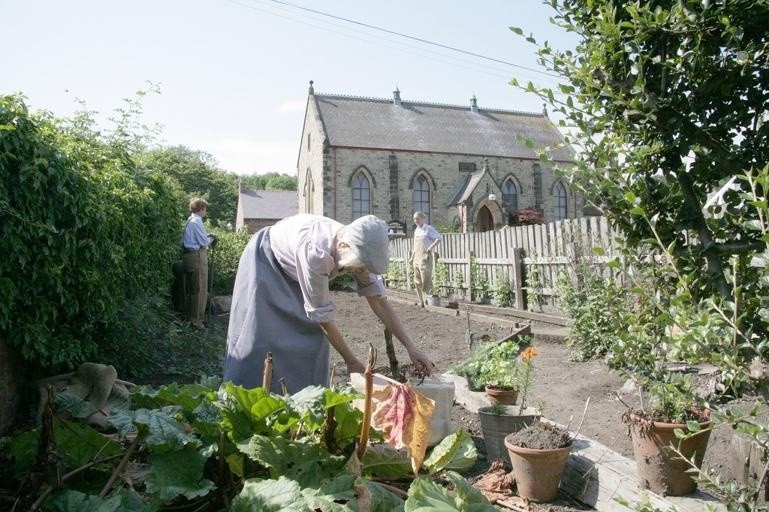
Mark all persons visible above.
[223,212,431,396]
[408,212,442,309]
[180,197,215,328]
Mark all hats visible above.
[349,214,390,275]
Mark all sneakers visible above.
[191,318,205,329]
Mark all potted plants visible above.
[468,333,536,405]
[540,228,733,493]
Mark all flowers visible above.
[517,346,538,415]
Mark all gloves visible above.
[207,234,220,248]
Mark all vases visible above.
[478,406,576,503]
